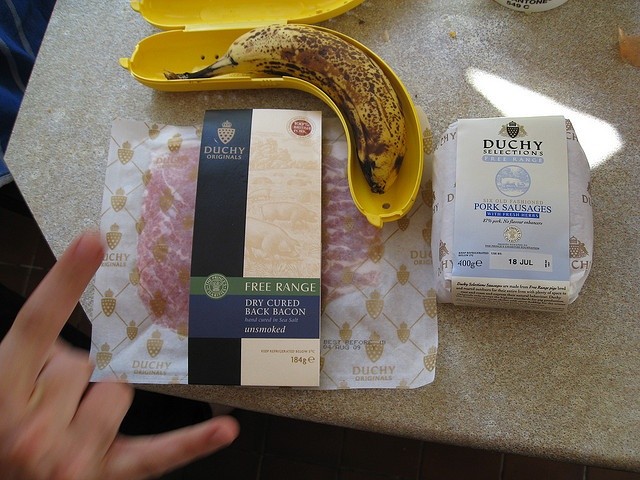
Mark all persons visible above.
[0,229,240,479]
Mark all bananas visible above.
[160,21,412,197]
